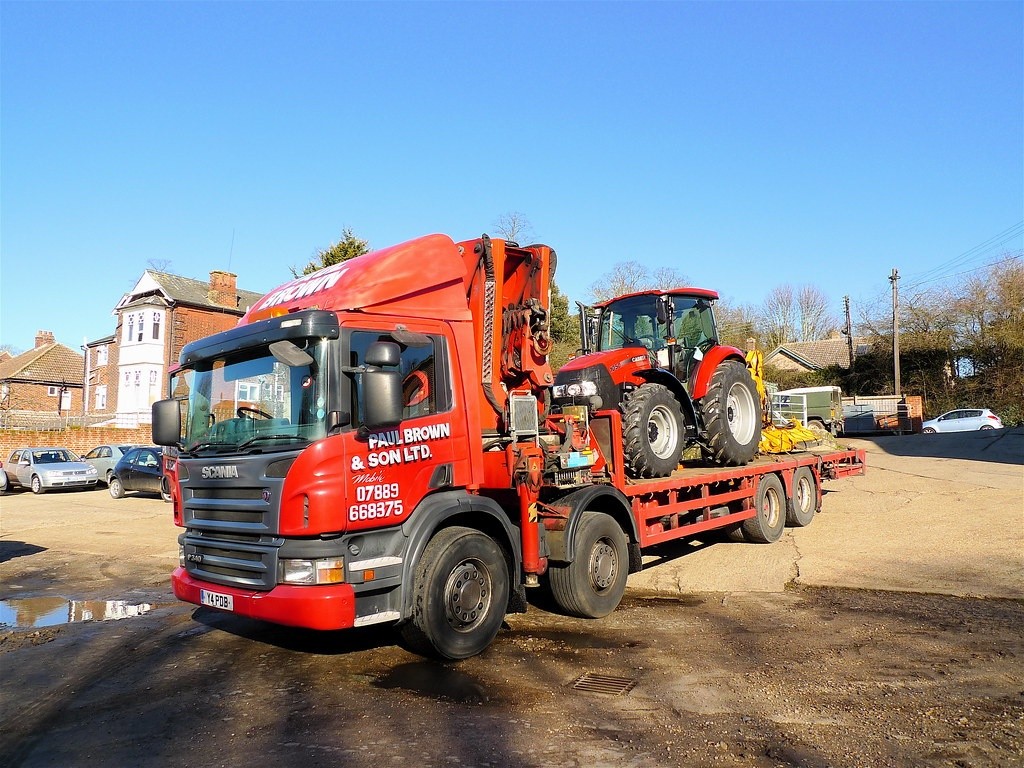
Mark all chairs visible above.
[145,454,157,466]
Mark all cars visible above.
[107,445,172,503]
[80,443,158,487]
[922,408,1004,434]
[4,447,99,494]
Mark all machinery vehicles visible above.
[550,286,762,480]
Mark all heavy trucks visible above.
[151,235,868,663]
